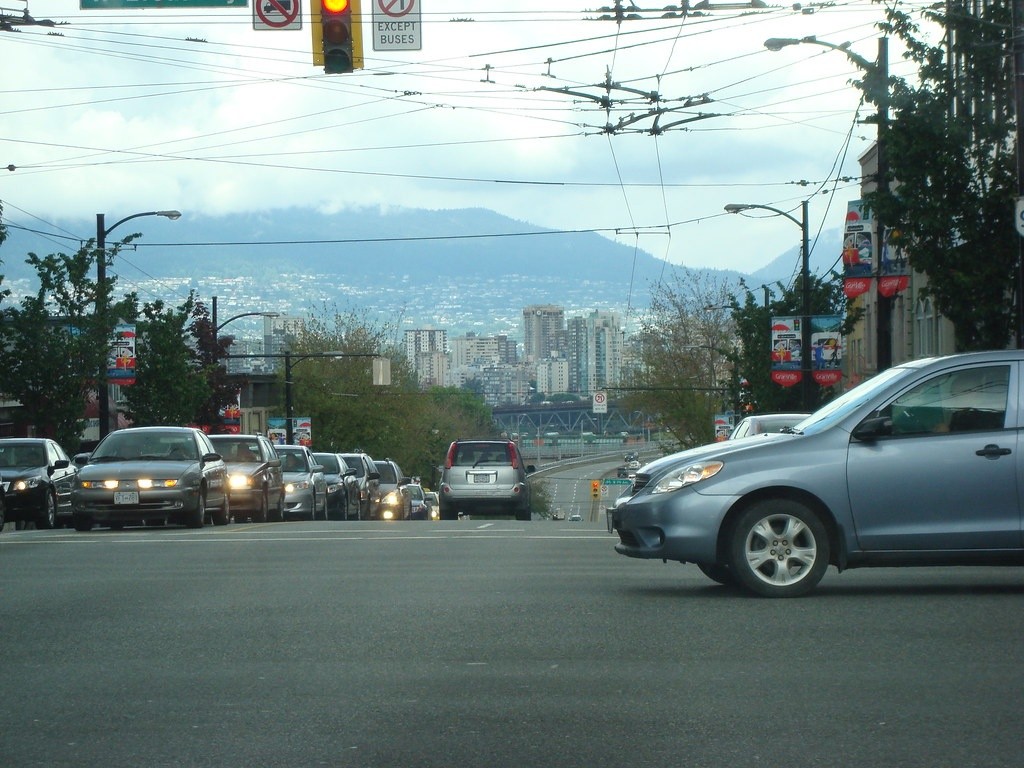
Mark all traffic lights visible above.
[591,479,600,499]
[310,0,364,75]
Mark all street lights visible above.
[722,199,811,409]
[211,296,280,435]
[284,349,345,444]
[762,36,897,374]
[96,210,181,443]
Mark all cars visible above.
[248,443,330,521]
[553,513,565,520]
[569,514,584,521]
[70,425,231,532]
[281,452,361,521]
[604,348,1024,601]
[337,453,381,521]
[0,438,94,531]
[405,475,440,520]
[616,451,642,479]
[726,413,813,441]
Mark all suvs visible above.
[373,457,413,520]
[439,437,537,521]
[186,431,286,523]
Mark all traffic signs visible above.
[593,390,607,413]
[372,0,422,51]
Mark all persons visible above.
[284,452,303,473]
[169,441,194,460]
[15,446,40,467]
[933,370,999,434]
[235,442,256,463]
